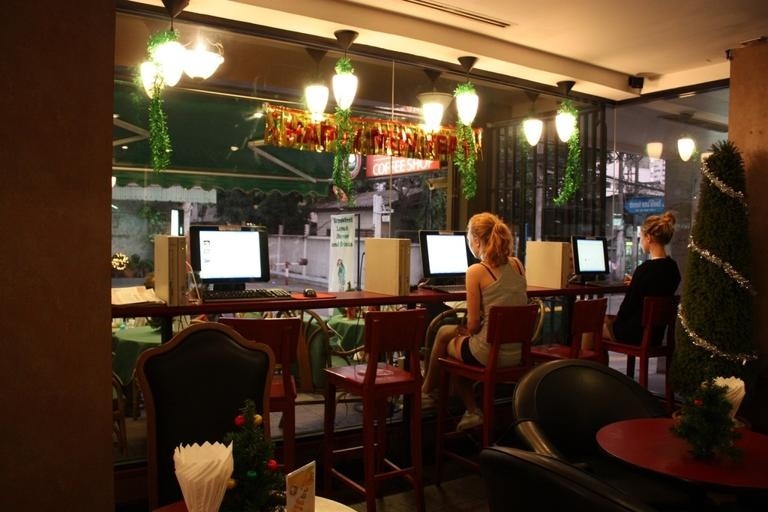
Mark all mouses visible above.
[303,288,316,297]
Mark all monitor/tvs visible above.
[569,235,610,284]
[189,225,271,291]
[170,208,184,236]
[416,230,472,287]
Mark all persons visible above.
[421,211,530,435]
[337,258,346,291]
[580,211,680,368]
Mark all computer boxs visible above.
[153,235,187,307]
[525,240,571,290]
[364,238,411,296]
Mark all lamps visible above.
[521,91,545,147]
[414,68,453,142]
[134,0,225,175]
[678,108,696,161]
[331,28,360,204]
[301,47,331,126]
[552,80,582,206]
[644,138,664,160]
[454,56,479,200]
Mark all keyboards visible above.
[438,286,466,293]
[588,279,627,288]
[202,287,291,303]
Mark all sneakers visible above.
[399,394,436,411]
[456,409,484,432]
